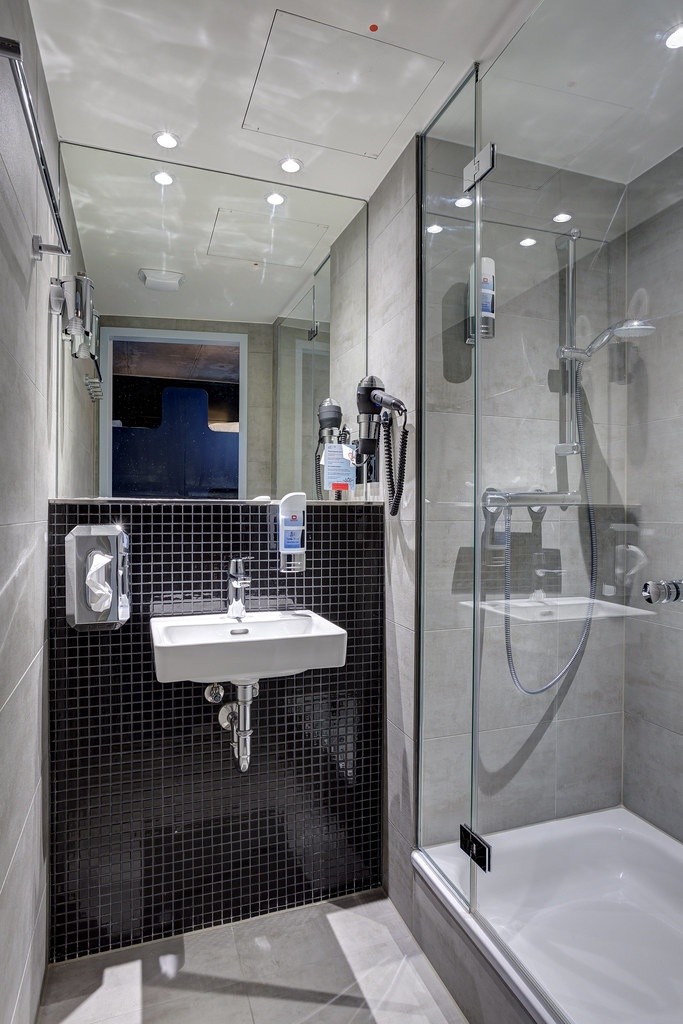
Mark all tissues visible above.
[64,524,130,632]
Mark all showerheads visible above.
[584,317,657,357]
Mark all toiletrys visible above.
[468,257,496,339]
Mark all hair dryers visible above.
[316,397,342,456]
[356,374,408,457]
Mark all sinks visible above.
[455,596,659,658]
[150,609,347,684]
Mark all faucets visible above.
[225,556,256,617]
[528,552,569,601]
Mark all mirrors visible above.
[46,136,371,500]
